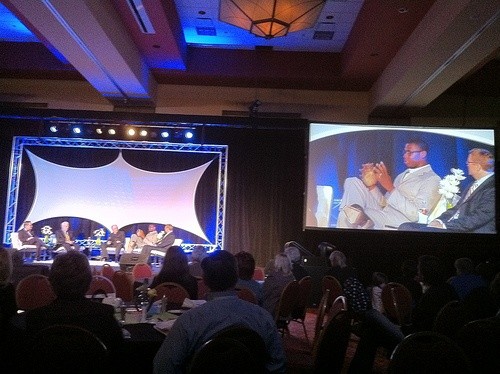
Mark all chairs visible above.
[106,237,183,267]
[317,185,333,226]
[274,241,500,374]
[24,324,108,374]
[10,233,50,261]
[190,324,267,374]
[15,274,56,311]
[51,234,85,254]
[86,261,264,309]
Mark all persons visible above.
[56,222,81,253]
[187,246,207,279]
[235,251,263,306]
[25,251,125,374]
[124,223,157,255]
[0,248,19,324]
[282,246,309,283]
[328,247,500,374]
[397,147,495,233]
[259,253,297,315]
[153,250,288,374]
[147,245,199,313]
[17,220,53,261]
[136,223,175,264]
[101,224,126,263]
[338,140,444,230]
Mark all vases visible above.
[96,237,101,246]
[44,235,48,242]
[445,199,453,210]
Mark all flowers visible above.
[41,225,53,235]
[92,228,105,236]
[438,168,466,202]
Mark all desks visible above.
[105,306,155,327]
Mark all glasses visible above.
[466,161,475,165]
[403,150,423,156]
